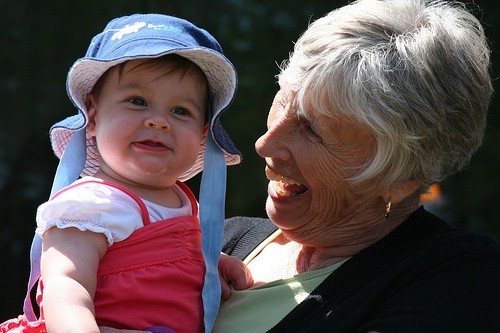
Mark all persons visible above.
[34,11,254,333]
[215,1,499,332]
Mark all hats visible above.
[49,12,244,184]
